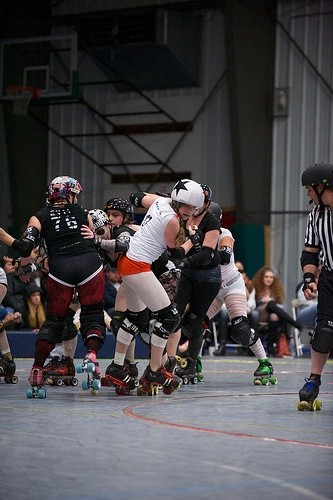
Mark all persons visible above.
[297,161,333,412]
[253,266,313,357]
[0,175,279,398]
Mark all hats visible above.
[26,285,44,300]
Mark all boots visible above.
[266,320,282,358]
[266,300,304,330]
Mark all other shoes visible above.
[213,341,225,356]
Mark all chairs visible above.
[256,320,287,355]
[291,283,318,356]
[211,318,248,353]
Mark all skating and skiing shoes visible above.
[163,355,183,375]
[175,357,205,385]
[137,361,183,396]
[0,355,18,384]
[76,349,102,390]
[43,355,79,386]
[254,358,278,386]
[101,359,138,386]
[297,373,323,411]
[26,365,46,399]
[105,358,141,395]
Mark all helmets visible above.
[302,160,333,190]
[198,184,212,204]
[49,176,83,201]
[208,202,222,222]
[171,179,205,207]
[88,208,109,230]
[104,197,131,216]
